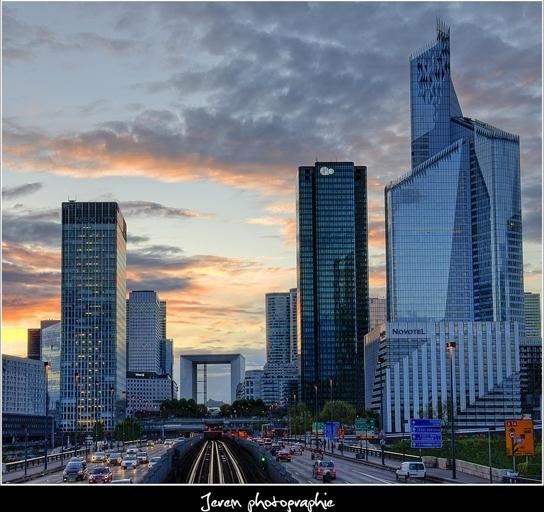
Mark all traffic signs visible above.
[505,420,534,457]
[410,419,442,448]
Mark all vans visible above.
[396,462,427,482]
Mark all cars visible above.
[63,437,186,484]
[246,437,336,483]
[501,471,518,483]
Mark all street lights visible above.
[110,387,148,449]
[75,372,79,457]
[377,358,384,465]
[95,381,99,452]
[269,377,333,454]
[44,361,49,470]
[446,342,457,479]
